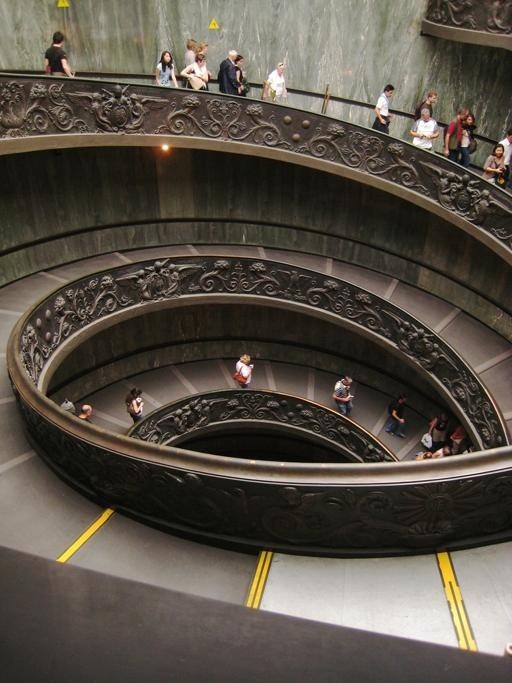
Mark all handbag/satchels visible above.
[469,139,477,154]
[127,404,143,417]
[233,372,247,385]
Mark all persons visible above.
[412,411,478,462]
[384,392,410,439]
[262,62,288,106]
[333,376,355,417]
[372,84,395,135]
[45,31,73,77]
[126,387,144,423]
[236,354,255,388]
[61,398,78,418]
[155,50,178,89]
[78,405,93,424]
[179,38,249,98]
[408,91,512,190]
